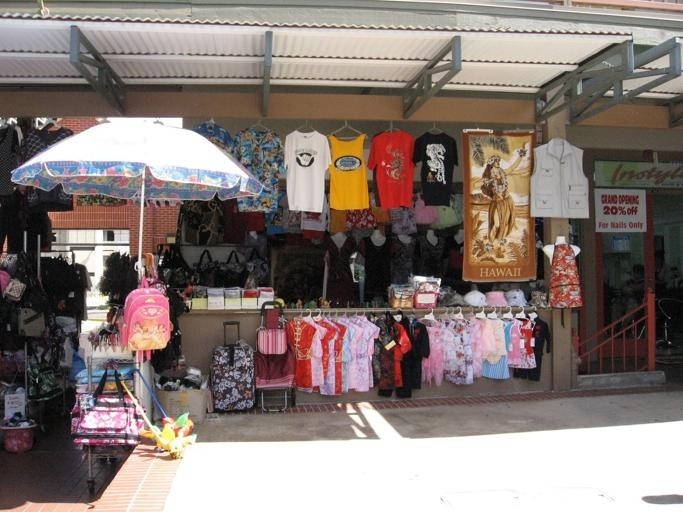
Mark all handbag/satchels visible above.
[0,251,46,336]
[157,248,268,287]
[70,363,144,446]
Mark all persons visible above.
[480,149,526,253]
[543,235,583,309]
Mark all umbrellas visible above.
[10,122,265,398]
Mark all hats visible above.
[444,289,548,308]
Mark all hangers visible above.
[203,119,447,141]
[291,307,541,327]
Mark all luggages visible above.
[255,301,296,412]
[210,321,255,411]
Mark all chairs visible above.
[642,290,680,350]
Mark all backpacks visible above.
[118,276,174,350]
